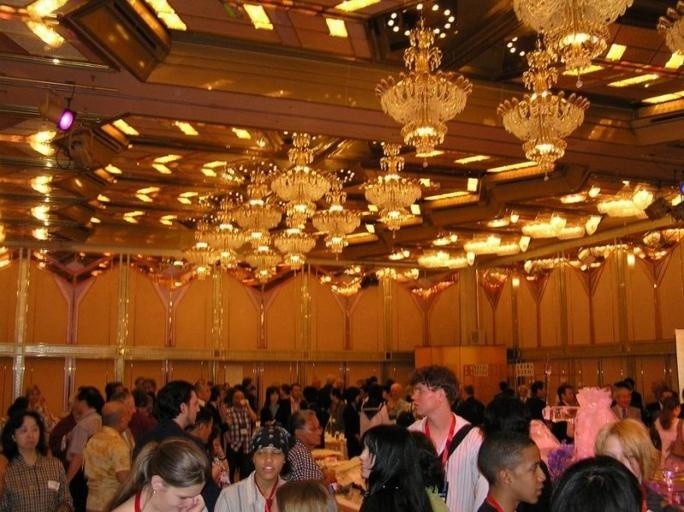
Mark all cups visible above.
[240,400,248,411]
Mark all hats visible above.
[248,428,289,472]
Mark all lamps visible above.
[37,87,77,133]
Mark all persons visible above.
[1,363,684,511]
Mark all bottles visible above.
[335,431,339,441]
